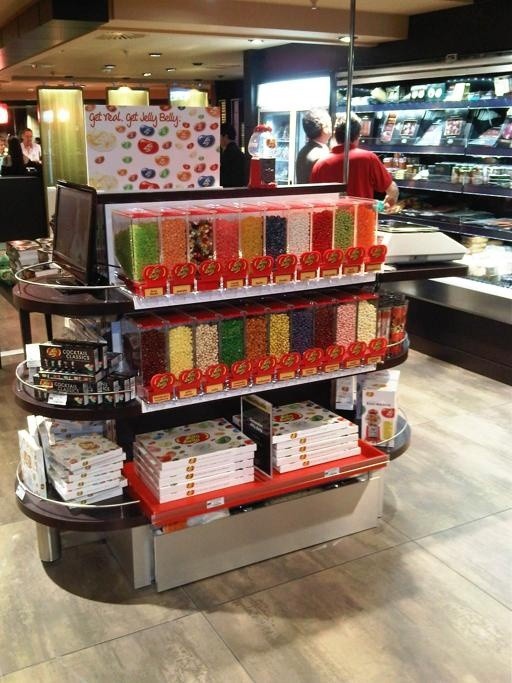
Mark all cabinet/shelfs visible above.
[9,182,411,596]
[256,108,310,185]
[331,54,512,324]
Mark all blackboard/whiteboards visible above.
[83,103,222,193]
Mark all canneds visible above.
[406,161,512,184]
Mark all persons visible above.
[219,121,249,188]
[19,128,45,162]
[0,133,9,174]
[3,134,44,176]
[309,109,399,210]
[35,137,41,144]
[297,106,332,183]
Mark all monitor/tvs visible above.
[44,180,98,295]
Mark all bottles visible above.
[452,166,512,188]
[382,154,426,174]
[275,125,289,179]
